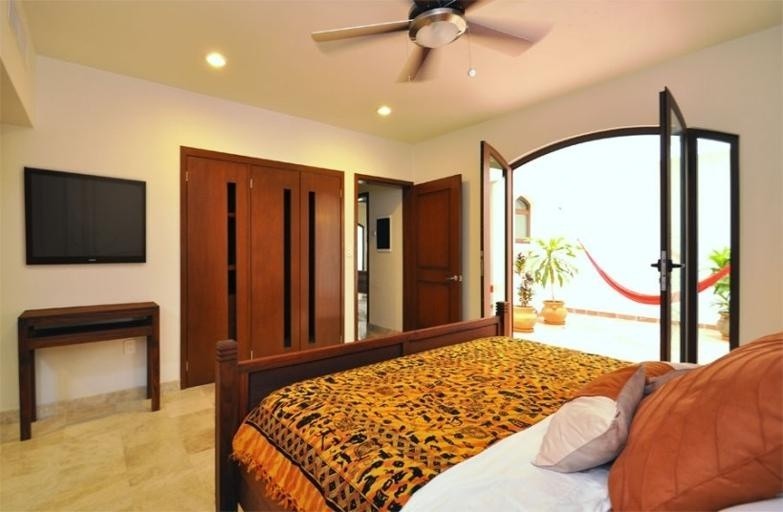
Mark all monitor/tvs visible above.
[24,167,146,265]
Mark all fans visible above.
[310,2,533,82]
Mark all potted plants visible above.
[711,250,731,343]
[513,253,538,334]
[537,235,578,324]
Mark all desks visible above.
[18,302,159,439]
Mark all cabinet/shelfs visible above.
[180,146,344,390]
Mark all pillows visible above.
[640,360,699,393]
[531,363,643,473]
[609,333,782,511]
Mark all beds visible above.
[215,302,783,512]
